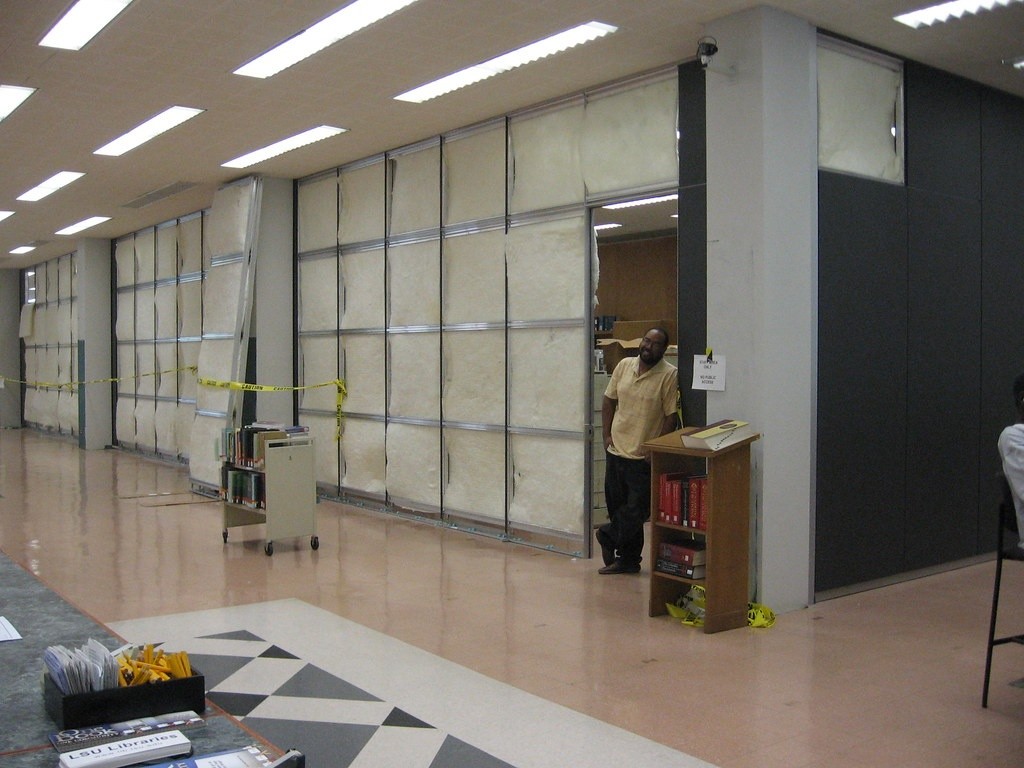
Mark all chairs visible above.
[982,471,1024,708]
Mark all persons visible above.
[997,373,1024,550]
[595,327,678,574]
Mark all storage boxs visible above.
[43,653,206,731]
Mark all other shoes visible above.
[596,528,615,566]
[598,560,641,574]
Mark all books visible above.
[654,538,706,580]
[219,425,310,510]
[657,470,708,531]
[680,419,755,451]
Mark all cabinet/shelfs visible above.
[218,422,319,556]
[641,426,760,634]
